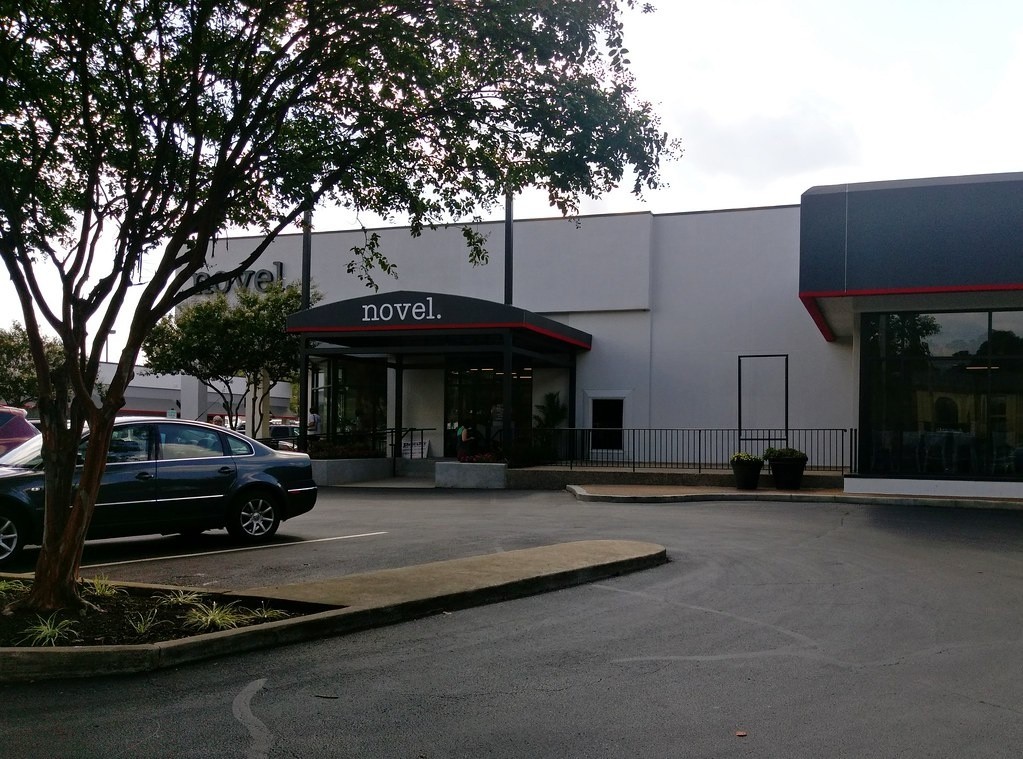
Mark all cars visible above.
[0,416,318,565]
[269,425,299,444]
[0,405,41,458]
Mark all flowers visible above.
[729,452,763,464]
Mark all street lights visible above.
[106,330,116,362]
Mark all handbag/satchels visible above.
[308,415,317,431]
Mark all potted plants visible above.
[763,447,809,491]
[530,392,568,461]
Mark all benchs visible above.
[177,438,224,456]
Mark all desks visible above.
[922,435,981,474]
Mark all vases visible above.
[732,464,763,490]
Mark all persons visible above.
[307,407,323,435]
[213,415,223,426]
[457,419,475,441]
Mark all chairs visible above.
[897,431,921,473]
[991,431,1017,476]
[107,439,140,464]
